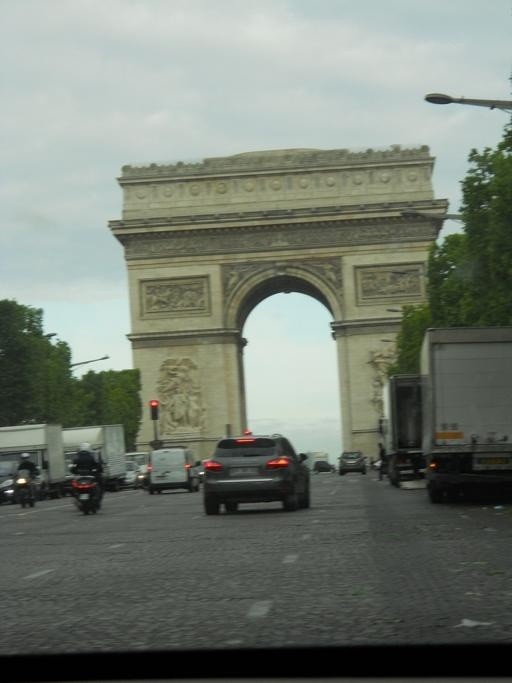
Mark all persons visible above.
[16,449,41,482]
[70,441,106,483]
[376,441,389,483]
[155,364,207,436]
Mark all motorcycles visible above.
[8,470,39,508]
[67,467,103,515]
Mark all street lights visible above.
[424,92,512,115]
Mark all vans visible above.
[149,447,199,495]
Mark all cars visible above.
[126,452,150,490]
[204,433,310,515]
[337,449,367,475]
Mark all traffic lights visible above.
[149,399,161,421]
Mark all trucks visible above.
[382,328,512,506]
[0,423,127,504]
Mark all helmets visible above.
[18,450,31,459]
[77,441,92,453]
